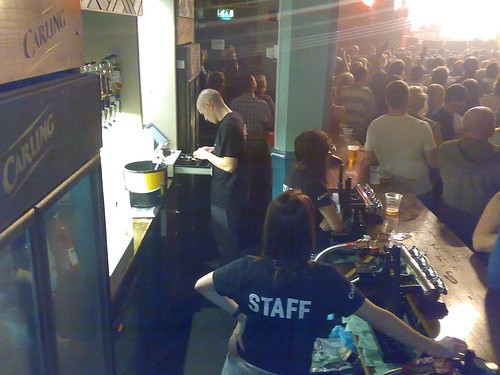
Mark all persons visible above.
[10,232,71,347]
[193,0,500,291]
[195,189,468,375]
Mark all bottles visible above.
[82,55,122,129]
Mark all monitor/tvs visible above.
[217,9,234,18]
[144,122,171,149]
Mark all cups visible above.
[368,165,382,185]
[342,128,353,141]
[411,231,434,257]
[339,123,348,137]
[348,145,360,160]
[316,338,344,359]
[384,192,403,216]
[380,166,393,184]
